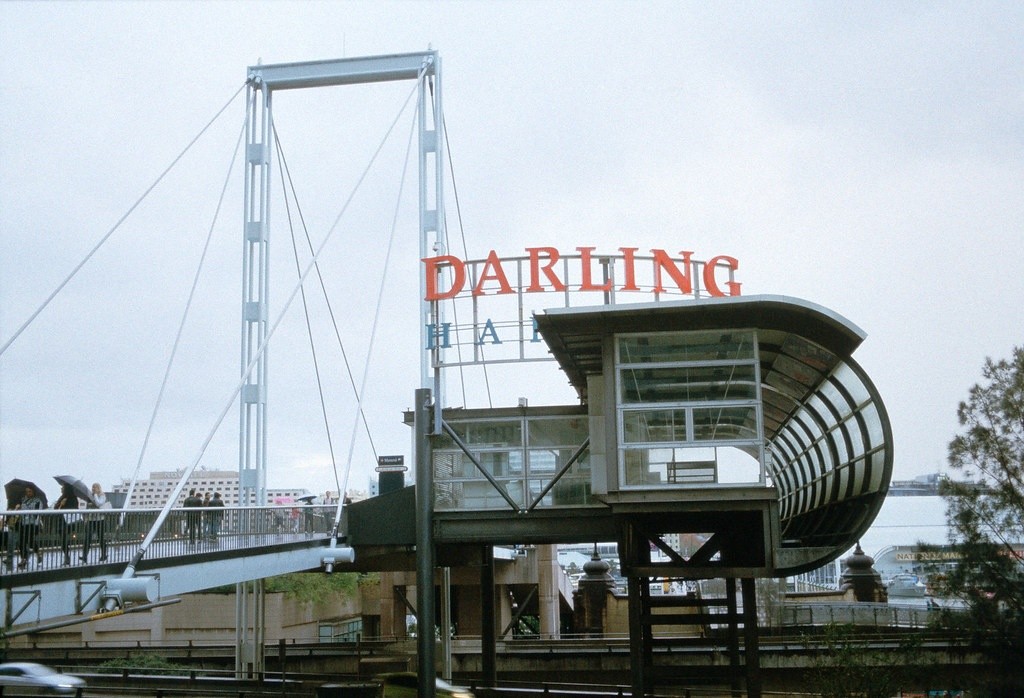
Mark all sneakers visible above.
[213,538,216,542]
[311,531,315,534]
[209,535,213,542]
[304,531,308,534]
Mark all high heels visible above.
[62,557,70,566]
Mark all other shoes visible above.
[17,559,28,567]
[99,556,108,562]
[37,552,43,564]
[78,556,88,564]
[189,540,195,544]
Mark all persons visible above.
[323,490,333,530]
[79,483,107,562]
[183,490,223,543]
[54,483,77,565]
[344,493,351,504]
[1,485,44,563]
[305,498,315,533]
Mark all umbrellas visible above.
[299,494,317,500]
[54,475,94,503]
[4,479,48,509]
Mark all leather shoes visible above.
[2,559,13,564]
